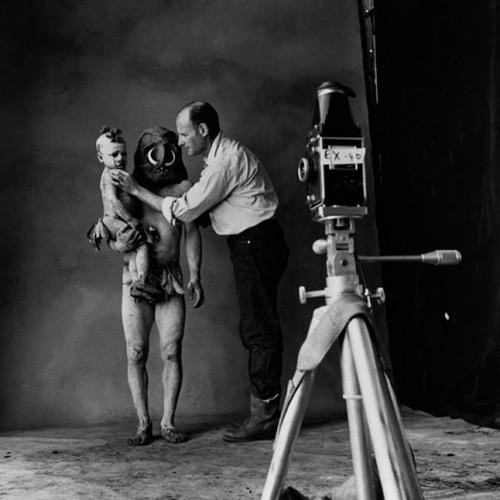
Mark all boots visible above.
[222,392,280,443]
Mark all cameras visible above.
[296,80,369,221]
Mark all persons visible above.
[88,125,207,448]
[95,125,166,305]
[104,98,293,443]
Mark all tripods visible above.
[260,222,425,499]
